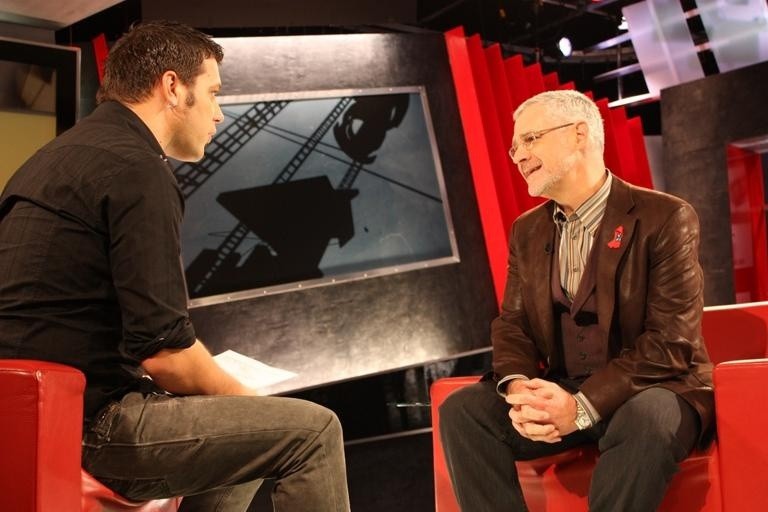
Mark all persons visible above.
[438,90,714,512]
[0,22,351,512]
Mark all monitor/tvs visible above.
[166,86,461,309]
[0,36,80,195]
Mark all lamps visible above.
[542,36,574,60]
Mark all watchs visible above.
[574,401,590,430]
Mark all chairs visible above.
[1,356,186,511]
[430,301,768,511]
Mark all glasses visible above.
[507,122,576,160]
